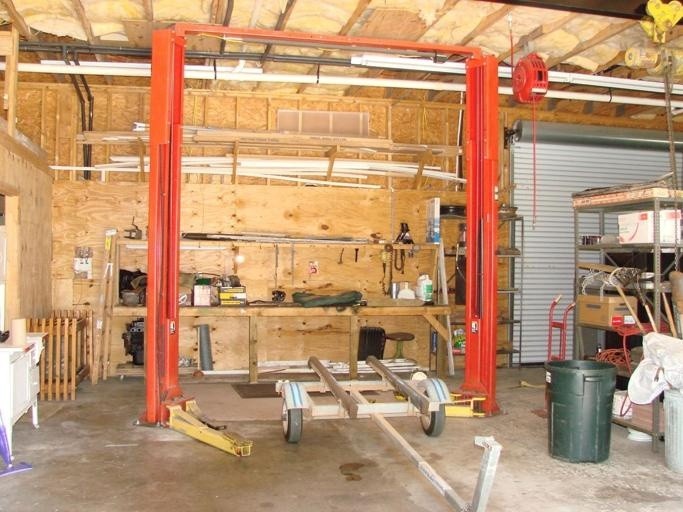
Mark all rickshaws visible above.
[275,355,503,512]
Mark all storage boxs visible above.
[617,209,681,245]
[576,294,639,328]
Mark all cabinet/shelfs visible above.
[430,215,523,374]
[572,182,683,453]
[0,332,49,464]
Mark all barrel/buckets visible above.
[416,274,432,302]
[544,359,617,463]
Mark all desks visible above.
[111,304,451,384]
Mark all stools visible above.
[386,333,417,359]
